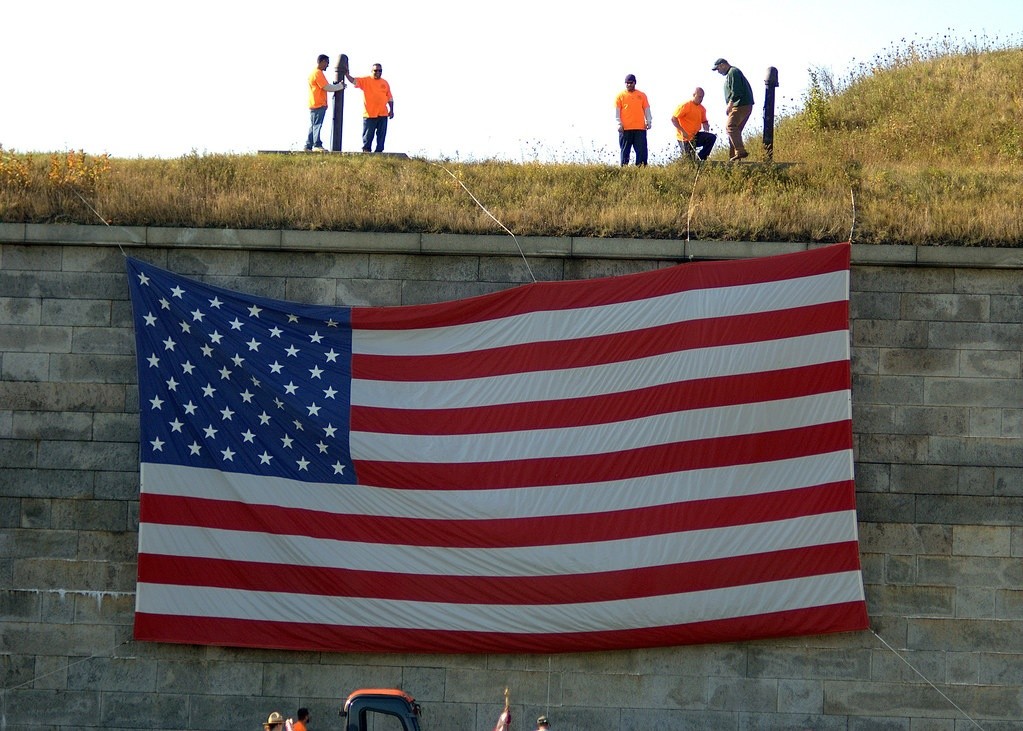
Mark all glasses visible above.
[373,69,382,72]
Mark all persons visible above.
[671,87,716,162]
[260,707,310,731]
[711,59,755,162]
[340,63,395,153]
[304,52,347,153]
[616,74,652,166]
[535,714,551,730]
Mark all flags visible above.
[126,245,873,656]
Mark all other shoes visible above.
[363,149,371,153]
[305,148,311,152]
[730,151,749,161]
[312,147,328,152]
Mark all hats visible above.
[262,712,284,724]
[712,59,727,71]
[537,716,548,723]
[625,74,637,84]
